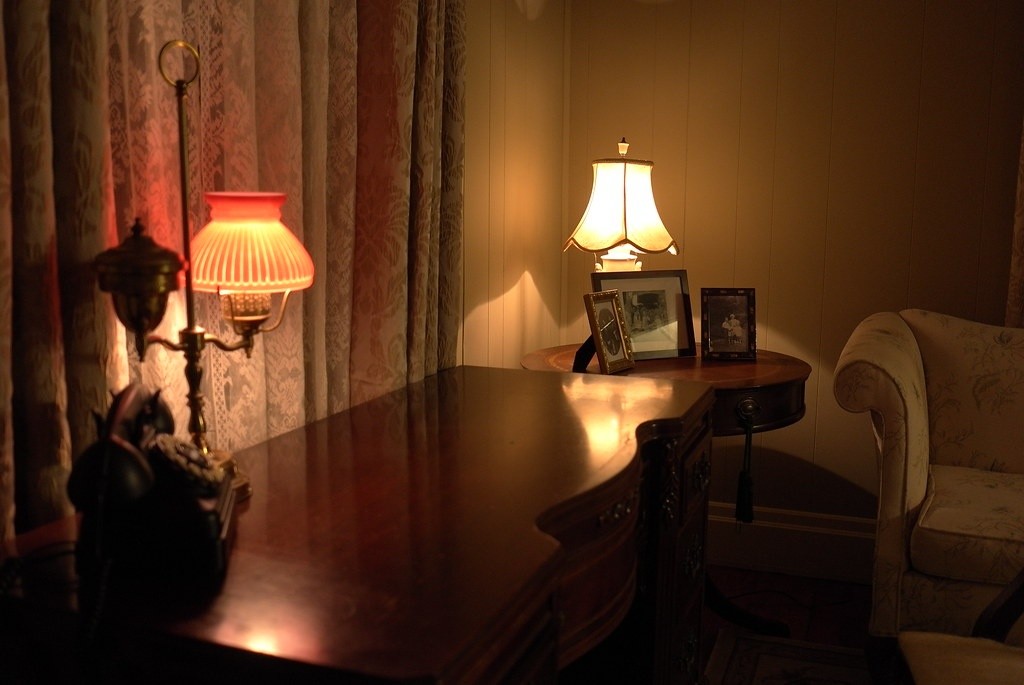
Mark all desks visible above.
[521,344,812,634]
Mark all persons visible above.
[602,320,611,345]
[731,314,742,343]
[722,317,733,344]
[629,302,665,331]
[607,316,620,353]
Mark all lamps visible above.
[563,137,679,272]
[95,41,316,504]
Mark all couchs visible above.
[833,309,1024,647]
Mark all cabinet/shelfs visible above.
[1,366,717,685]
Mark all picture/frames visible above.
[700,287,756,362]
[591,270,697,361]
[572,289,636,375]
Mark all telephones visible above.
[66,380,240,615]
